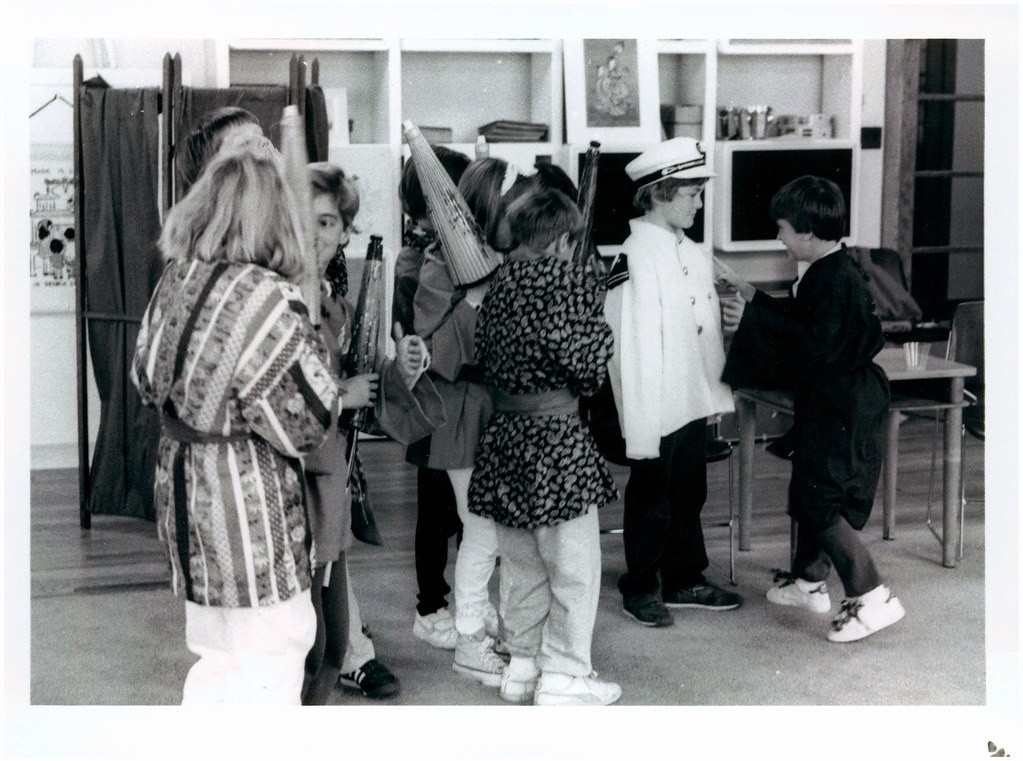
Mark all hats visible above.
[623,135,719,188]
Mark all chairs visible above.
[590,369,732,573]
[869,248,953,344]
[926,301,985,561]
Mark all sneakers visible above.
[825,582,906,643]
[501,665,537,702]
[339,659,401,699]
[622,594,674,626]
[662,582,744,611]
[764,578,831,614]
[412,607,460,651]
[452,635,507,688]
[535,671,621,706]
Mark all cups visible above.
[674,106,702,138]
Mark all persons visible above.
[723,178,905,641]
[131,109,446,705]
[393,146,622,706]
[603,138,743,626]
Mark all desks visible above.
[735,348,977,568]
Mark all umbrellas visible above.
[280,105,321,330]
[345,235,386,485]
[401,119,502,287]
[573,142,603,266]
